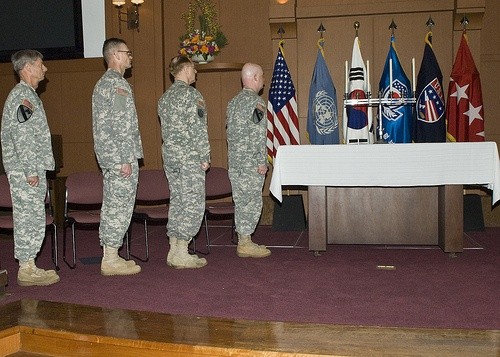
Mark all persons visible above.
[225,63,271,258]
[158,53,211,269]
[92,37,144,276]
[1,50,59,286]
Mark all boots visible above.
[166,236,198,266]
[237,234,266,254]
[101,244,136,275]
[18,259,60,286]
[237,236,271,258]
[102,246,141,276]
[17,258,56,283]
[172,240,208,269]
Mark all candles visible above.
[345,57,416,93]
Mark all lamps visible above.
[112,0,145,34]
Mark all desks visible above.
[269,142,500,257]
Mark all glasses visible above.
[114,51,132,56]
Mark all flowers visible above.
[177,0,230,61]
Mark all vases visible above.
[192,54,214,65]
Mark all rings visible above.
[128,174,130,177]
[29,182,31,185]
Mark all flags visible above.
[377,37,415,144]
[445,30,485,142]
[343,38,375,144]
[411,32,446,143]
[267,39,300,166]
[306,39,340,144]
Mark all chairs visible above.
[0,174,59,272]
[63,167,238,269]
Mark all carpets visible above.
[0,223,500,330]
